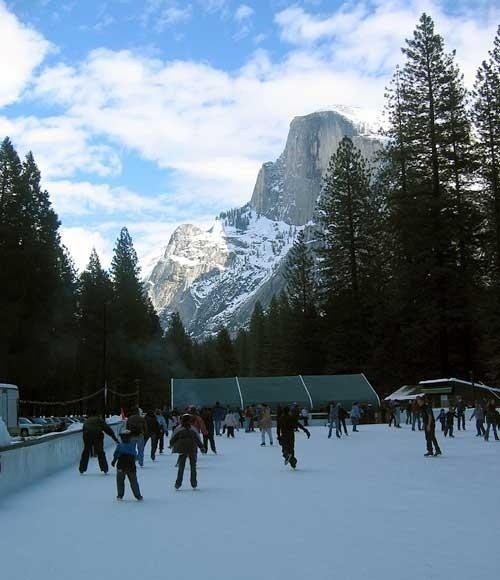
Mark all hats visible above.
[17,413,119,438]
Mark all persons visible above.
[258,403,273,446]
[350,401,361,432]
[453,398,466,430]
[288,402,309,433]
[162,407,190,430]
[169,414,205,491]
[470,399,498,440]
[80,414,120,475]
[417,398,441,456]
[367,399,402,428]
[213,401,225,435]
[277,407,310,470]
[436,409,447,431]
[155,411,168,455]
[406,400,424,431]
[125,408,147,466]
[197,406,217,453]
[143,410,161,463]
[440,407,458,438]
[189,407,207,453]
[328,402,350,438]
[222,405,243,438]
[111,423,145,500]
[245,405,256,432]
